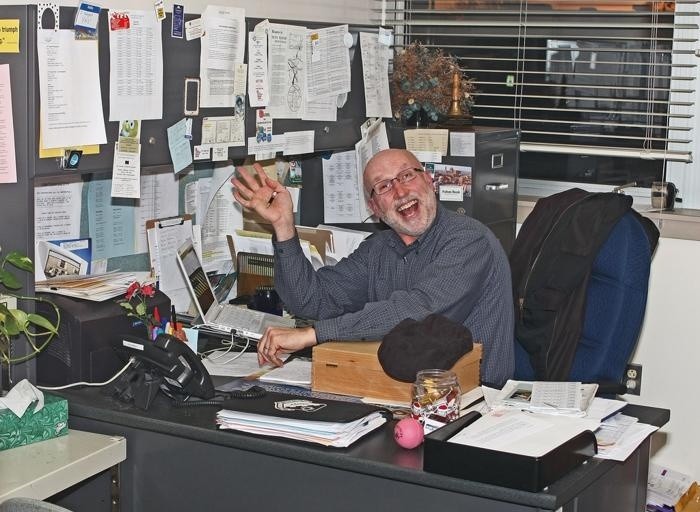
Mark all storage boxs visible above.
[311,341,482,403]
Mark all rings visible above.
[251,193,256,200]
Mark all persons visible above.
[231,147,516,387]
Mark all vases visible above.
[146,325,163,340]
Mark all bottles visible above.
[412,368,461,434]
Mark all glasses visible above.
[369,168,425,199]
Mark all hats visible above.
[377,314,473,383]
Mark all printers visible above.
[34,286,172,391]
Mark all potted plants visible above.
[392,40,482,128]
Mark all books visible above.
[491,379,599,418]
[260,359,312,390]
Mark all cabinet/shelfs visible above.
[300,125,520,260]
[35,292,171,387]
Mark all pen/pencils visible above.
[172,305,177,331]
[267,164,290,208]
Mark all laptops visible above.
[176,235,297,343]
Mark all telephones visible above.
[114,333,215,400]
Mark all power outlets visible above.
[621,364,642,397]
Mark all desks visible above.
[35,370,670,512]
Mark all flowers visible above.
[113,281,164,328]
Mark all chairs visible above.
[511,208,651,401]
